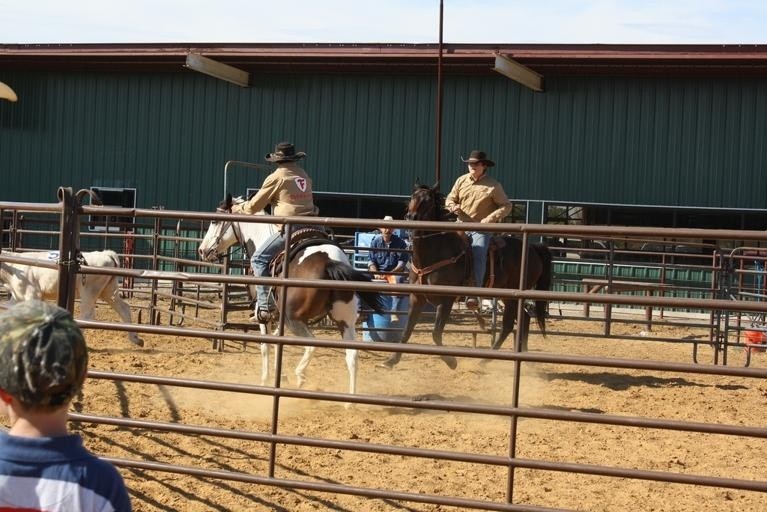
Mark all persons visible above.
[230,141,319,323]
[443,150,513,310]
[0,297,130,512]
[353,214,412,324]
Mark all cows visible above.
[0,248,144,348]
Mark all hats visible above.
[264,140,307,163]
[0,296,87,395]
[459,150,496,168]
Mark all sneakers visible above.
[465,296,479,307]
[390,314,400,324]
[248,310,280,323]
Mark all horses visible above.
[197,191,385,410]
[375,175,553,371]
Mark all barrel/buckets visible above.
[361,280,412,346]
